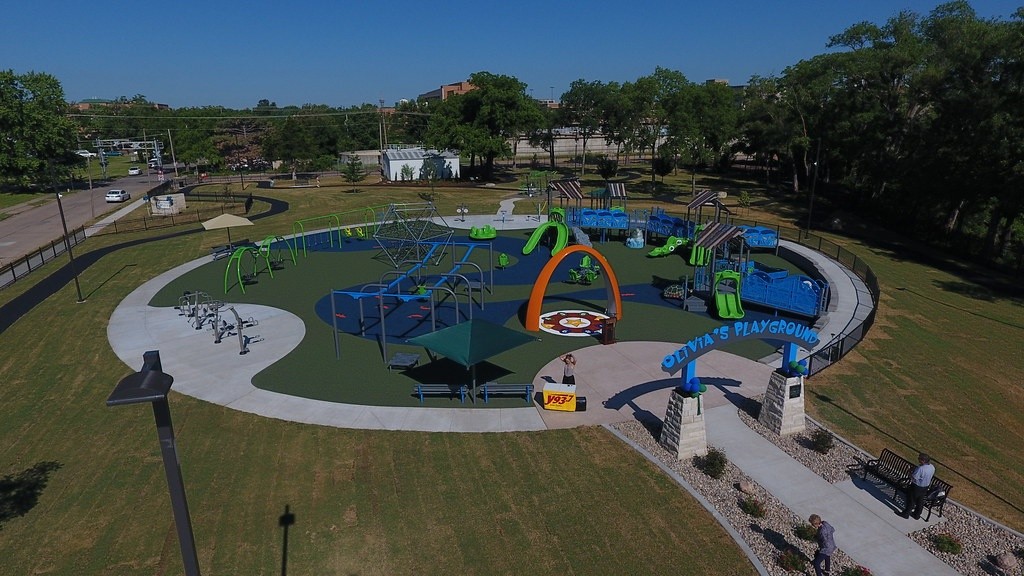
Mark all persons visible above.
[897,452,935,520]
[808,514,837,576]
[561,354,576,385]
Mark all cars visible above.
[105,189,131,203]
[128,166,142,176]
[101,150,123,157]
[133,149,158,169]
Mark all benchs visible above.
[414,384,469,403]
[863,448,953,522]
[232,239,254,250]
[209,245,231,261]
[480,381,534,403]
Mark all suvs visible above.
[75,149,97,160]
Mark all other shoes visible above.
[910,511,919,519]
[899,510,909,519]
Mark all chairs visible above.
[499,253,509,271]
[567,255,602,285]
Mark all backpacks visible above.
[926,484,946,505]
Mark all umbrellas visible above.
[201,213,255,242]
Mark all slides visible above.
[551,223,568,257]
[649,236,686,256]
[689,235,710,266]
[522,223,550,255]
[715,289,745,319]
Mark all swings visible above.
[270,244,282,264]
[240,259,254,280]
[307,219,329,247]
[343,211,365,238]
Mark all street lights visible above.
[104,349,203,576]
